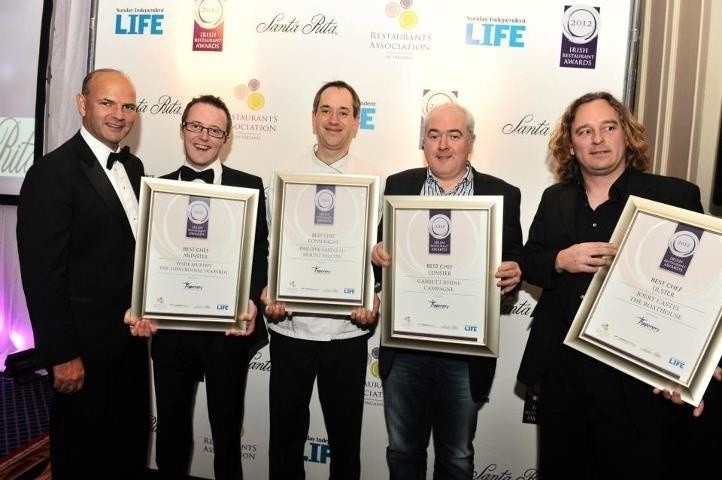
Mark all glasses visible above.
[183,122,228,139]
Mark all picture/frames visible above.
[561,191,722,408]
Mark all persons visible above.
[256,81,382,480]
[122,94,269,480]
[523,92,719,480]
[16,68,151,479]
[371,103,522,480]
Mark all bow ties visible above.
[107,145,130,170]
[181,166,214,184]
